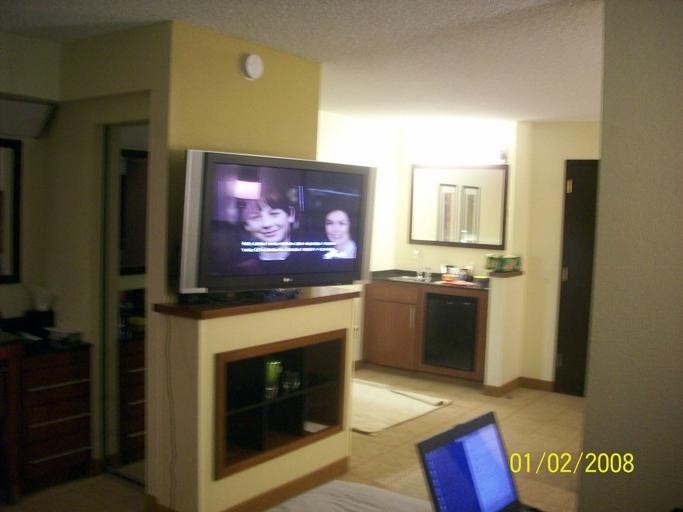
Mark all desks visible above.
[0,330,24,505]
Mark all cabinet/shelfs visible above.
[151,285,363,512]
[363,281,419,373]
[21,348,92,493]
[121,337,145,464]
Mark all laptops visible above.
[417,411,544,512]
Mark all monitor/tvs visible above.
[166,149,378,307]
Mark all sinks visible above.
[386,275,432,284]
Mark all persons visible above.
[230,181,305,275]
[321,201,359,259]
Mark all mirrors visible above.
[409,164,509,250]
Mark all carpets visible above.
[260,478,433,512]
[351,378,454,434]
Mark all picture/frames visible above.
[459,185,480,243]
[437,183,457,242]
[0,136,22,283]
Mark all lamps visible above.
[241,54,264,81]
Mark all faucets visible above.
[413,249,422,276]
